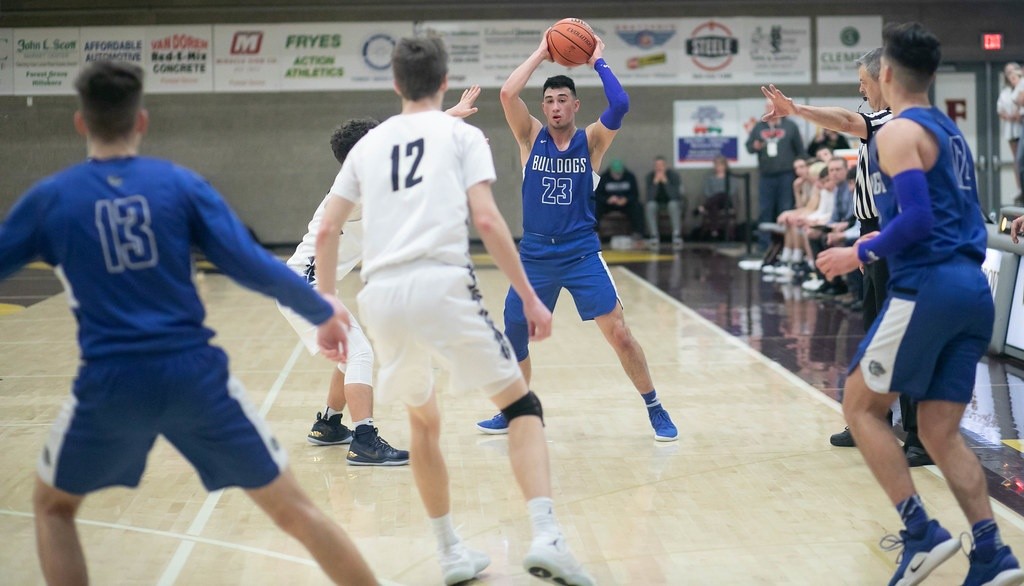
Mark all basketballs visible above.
[547,17,597,68]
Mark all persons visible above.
[691,155,739,242]
[745,99,804,250]
[645,155,683,243]
[0,58,381,586]
[274,84,490,465]
[995,61,1024,244]
[807,126,850,157]
[594,159,642,238]
[762,47,935,466]
[815,22,1024,586]
[764,154,863,311]
[476,27,678,442]
[314,37,593,586]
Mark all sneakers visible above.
[877,518,961,586]
[900,437,937,467]
[648,408,678,441]
[830,410,893,447]
[346,430,410,465]
[307,412,353,444]
[961,544,1024,586]
[522,540,593,586]
[439,546,490,586]
[477,412,510,434]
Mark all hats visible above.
[610,159,624,172]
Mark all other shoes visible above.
[673,237,683,244]
[649,237,659,244]
[737,252,865,310]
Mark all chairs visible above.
[603,191,742,241]
